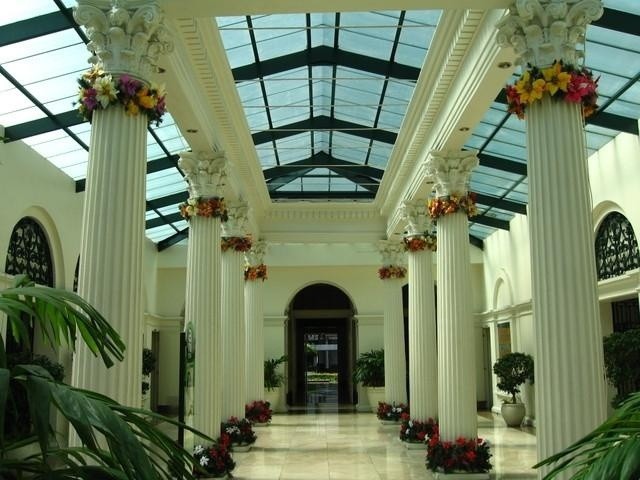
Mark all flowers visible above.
[72,61,166,127]
[220,234,253,252]
[178,196,228,222]
[504,58,601,118]
[378,400,493,475]
[397,230,437,252]
[243,263,268,282]
[426,189,478,217]
[192,400,272,478]
[376,263,408,279]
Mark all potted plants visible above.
[494,352,535,426]
[263,355,289,415]
[350,346,386,412]
